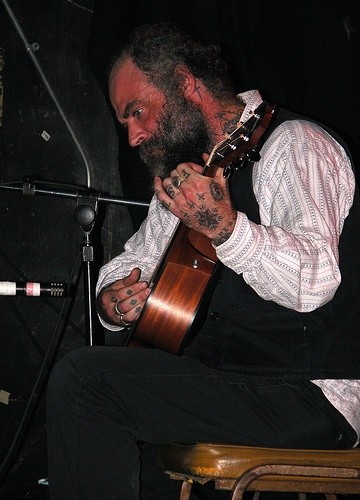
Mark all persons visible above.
[45,22,360,499]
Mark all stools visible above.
[160,442,360,500]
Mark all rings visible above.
[114,300,125,316]
[173,177,184,189]
[120,315,131,326]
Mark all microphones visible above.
[0,280,67,298]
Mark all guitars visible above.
[127,102,274,356]
